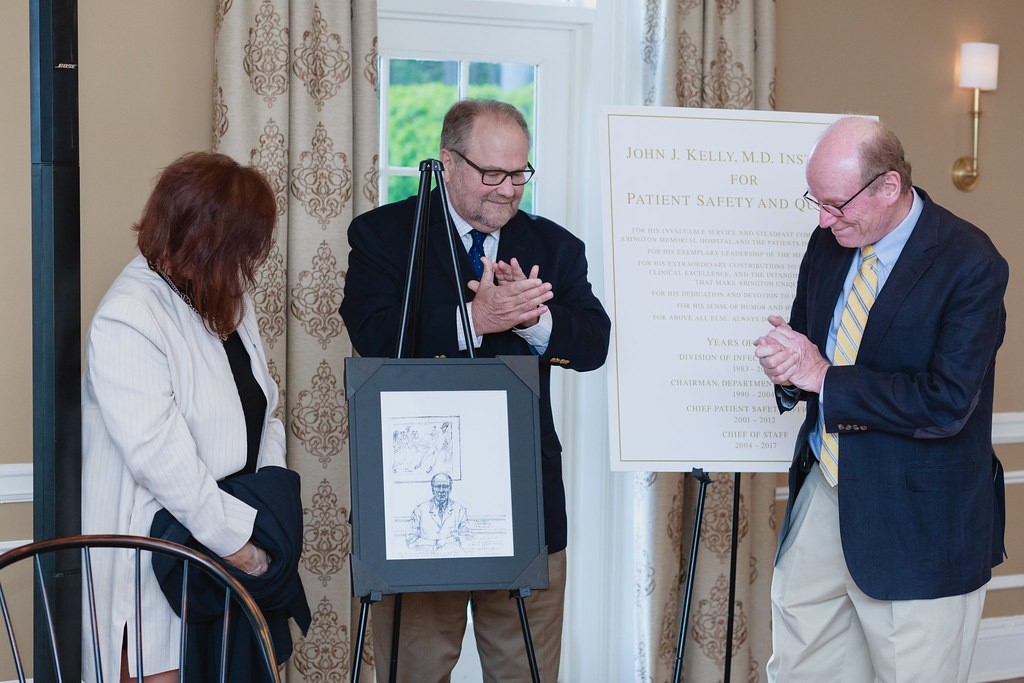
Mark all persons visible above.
[338,98,611,683]
[88,151,289,683]
[755,116,1011,683]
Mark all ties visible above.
[468,228,490,281]
[819,245,878,488]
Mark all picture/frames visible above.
[342,355,549,598]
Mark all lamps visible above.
[951,42,1000,193]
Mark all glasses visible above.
[803,171,886,217]
[449,148,536,186]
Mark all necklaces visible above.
[156,262,227,341]
